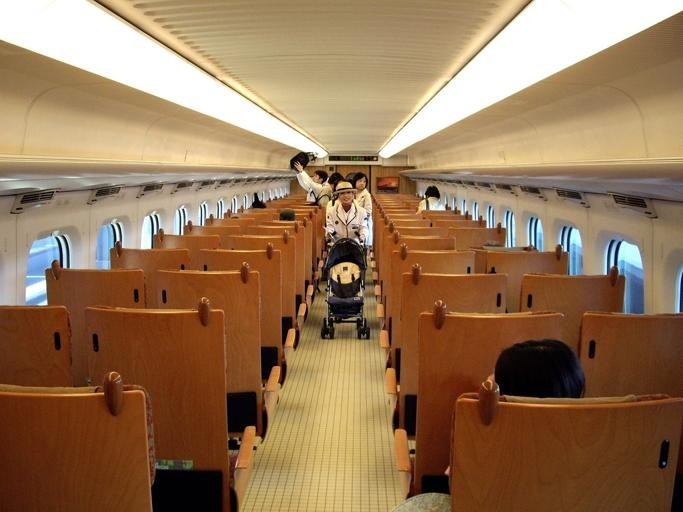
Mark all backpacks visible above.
[307,186,330,205]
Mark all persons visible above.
[294,160,374,255]
[417,186,446,215]
[278,207,296,220]
[444,339,585,474]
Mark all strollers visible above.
[318,237,370,339]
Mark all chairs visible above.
[1,193,326,512]
[371,192,683,512]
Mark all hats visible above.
[333,181,358,195]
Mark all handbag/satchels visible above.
[289,151,310,171]
